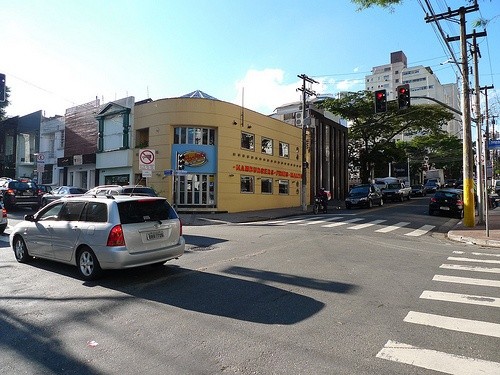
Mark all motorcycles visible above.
[312,195,328,214]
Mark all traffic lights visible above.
[0,73,6,102]
[176,151,185,172]
[397,84,411,109]
[304,162,309,170]
[375,90,387,113]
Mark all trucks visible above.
[426,169,445,190]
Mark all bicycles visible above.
[487,185,496,209]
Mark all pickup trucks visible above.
[382,183,412,203]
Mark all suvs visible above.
[345,182,383,210]
[0,178,158,234]
[9,194,185,281]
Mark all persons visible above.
[318,188,328,214]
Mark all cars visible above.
[428,184,476,218]
[410,179,463,197]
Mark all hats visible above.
[320,188,324,191]
